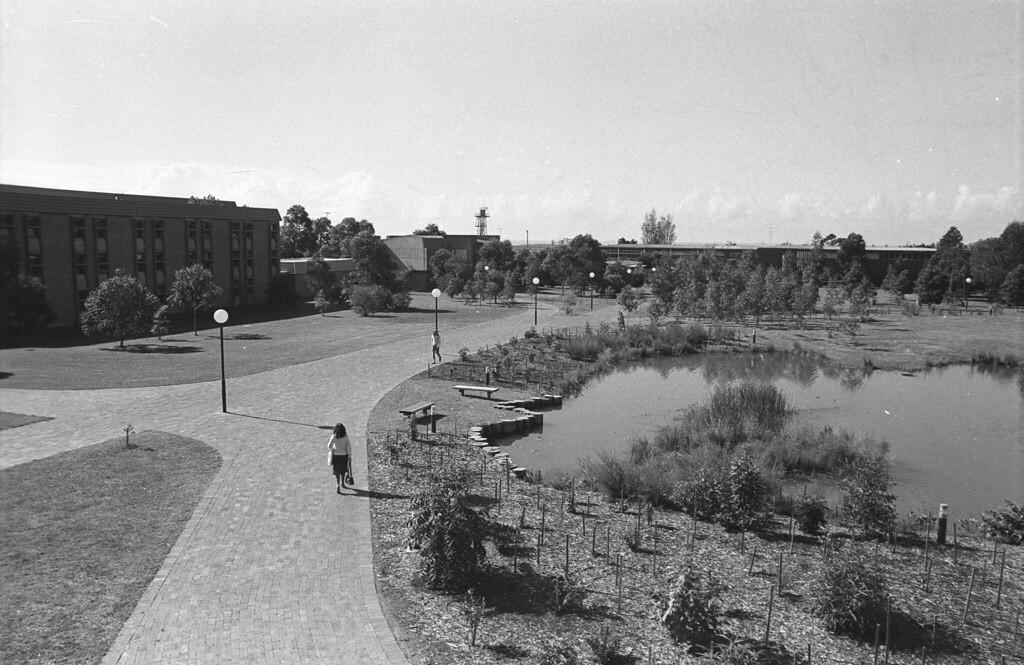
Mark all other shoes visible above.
[336,487,342,494]
[432,361,436,364]
[340,482,345,487]
[439,357,442,361]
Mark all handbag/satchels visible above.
[344,472,354,485]
[327,450,334,466]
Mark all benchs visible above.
[453,384,499,399]
[398,401,435,421]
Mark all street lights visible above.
[627,269,632,285]
[432,288,442,329]
[966,277,972,309]
[533,277,540,325]
[214,309,231,411]
[589,272,597,310]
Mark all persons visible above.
[431,330,442,364]
[328,423,352,494]
